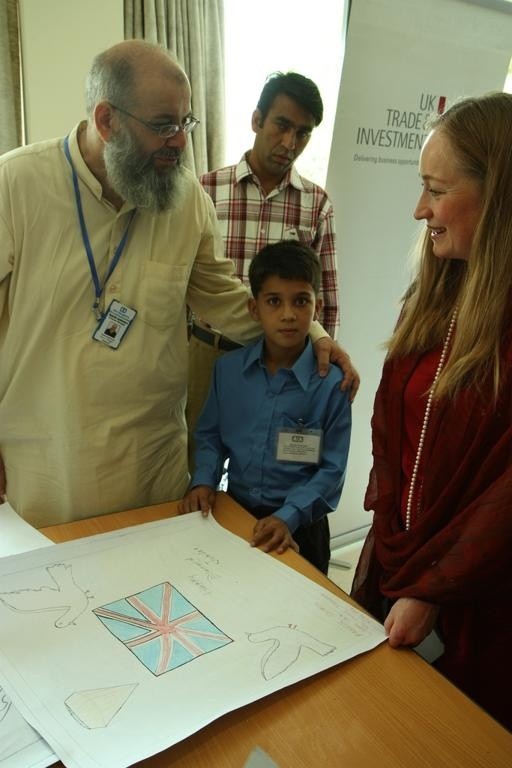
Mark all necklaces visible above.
[406,266,466,534]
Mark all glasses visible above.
[108,102,201,139]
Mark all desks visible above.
[38,491,511,766]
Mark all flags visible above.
[90,582,234,677]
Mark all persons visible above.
[187,72,342,484]
[1,41,360,529]
[177,240,352,577]
[351,93,512,732]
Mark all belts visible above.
[190,323,243,351]
